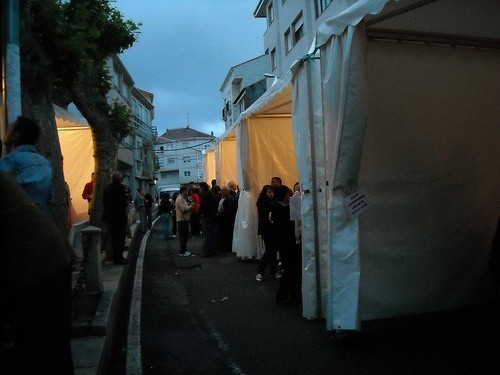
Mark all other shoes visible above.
[256,273,263,281]
[278,300,292,306]
[274,273,283,279]
[179,251,191,256]
[201,254,209,258]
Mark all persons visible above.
[101,171,132,265]
[82,173,302,305]
[0,170,78,375]
[0,117,54,210]
